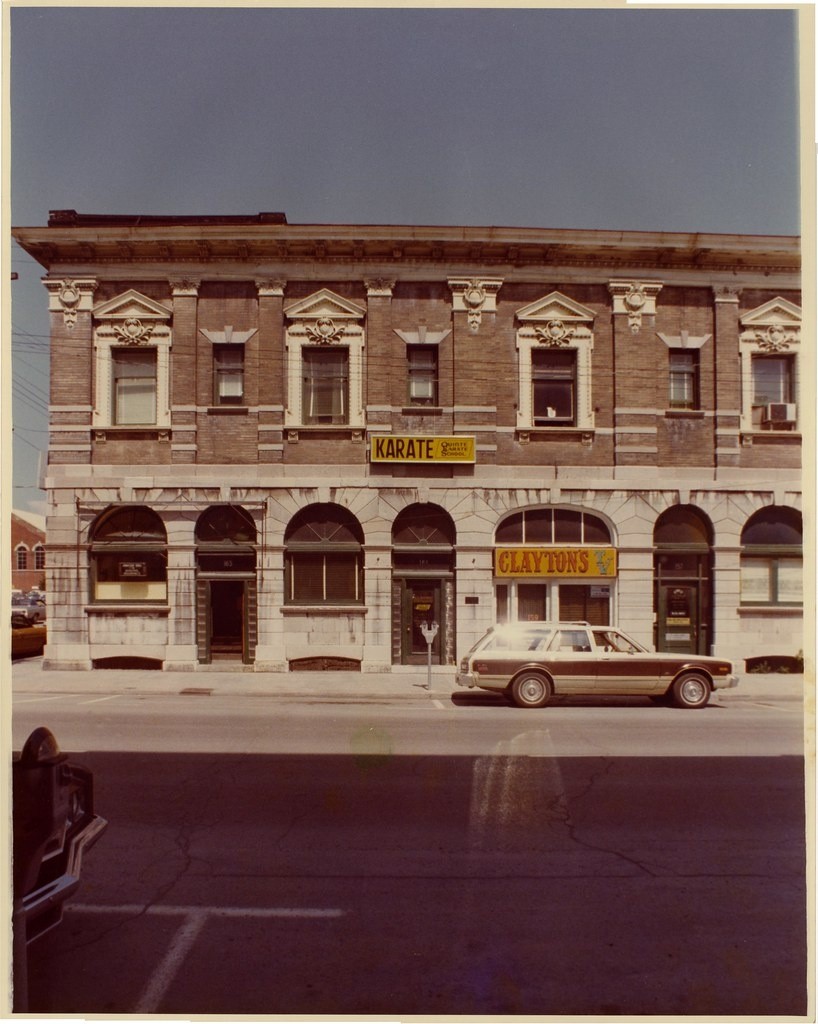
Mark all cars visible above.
[456,620,741,710]
[12,598,46,620]
[11,614,48,659]
